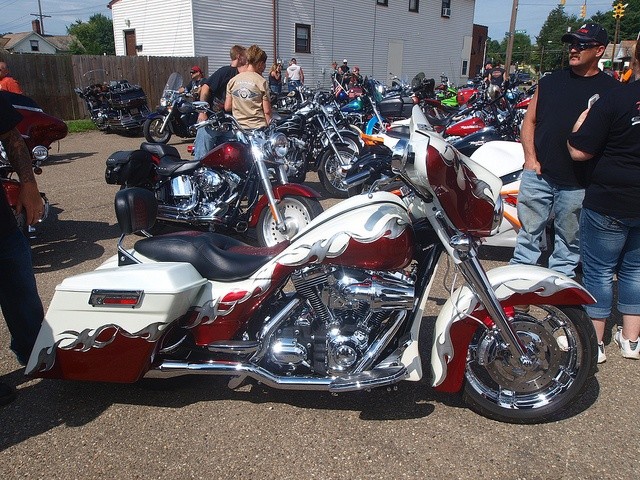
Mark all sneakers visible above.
[557,336,606,364]
[614,326,640,359]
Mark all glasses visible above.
[569,43,605,50]
[190,71,197,73]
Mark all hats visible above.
[343,59,347,62]
[192,66,203,76]
[561,23,609,47]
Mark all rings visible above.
[39,210,43,214]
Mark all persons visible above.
[193,46,248,158]
[176,66,207,100]
[482,61,493,86]
[228,44,247,67]
[338,58,350,85]
[603,59,618,80]
[285,58,304,92]
[224,45,273,212]
[329,62,339,79]
[1,58,24,100]
[268,60,284,96]
[566,34,640,364]
[510,23,621,280]
[343,66,363,87]
[488,62,506,97]
[0,90,45,366]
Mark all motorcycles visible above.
[24,104,598,424]
[0,135,51,233]
[74,69,153,135]
[376,72,538,164]
[269,70,393,198]
[105,110,324,247]
[142,71,199,144]
[469,140,554,251]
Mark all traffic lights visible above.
[579,5,587,18]
[612,4,625,19]
